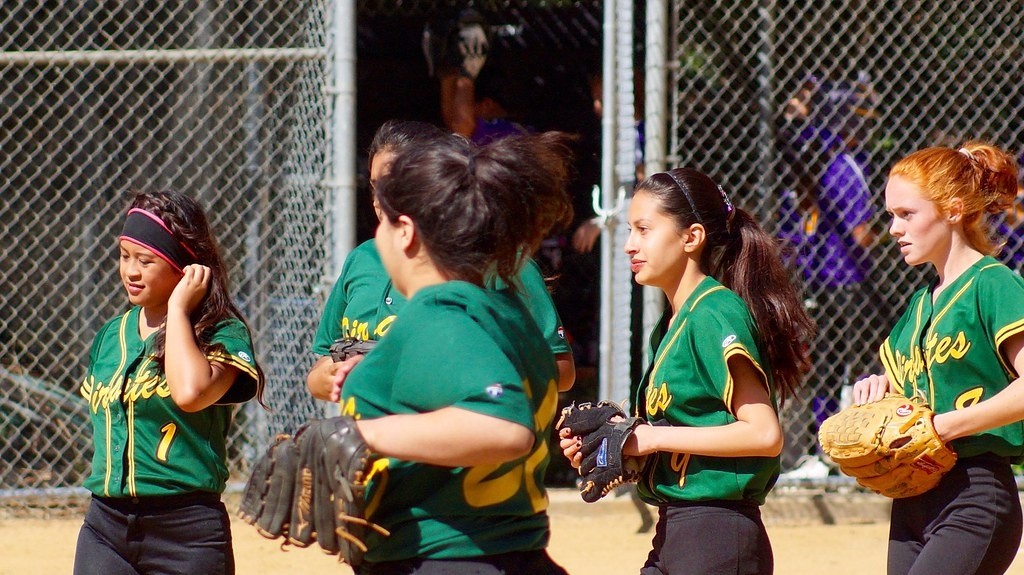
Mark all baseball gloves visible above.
[234,405,389,567]
[815,390,960,503]
[557,398,652,503]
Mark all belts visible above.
[97,491,221,509]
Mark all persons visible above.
[235,117,583,575]
[555,165,820,575]
[817,141,1024,574]
[71,188,276,574]
[436,70,1024,487]
